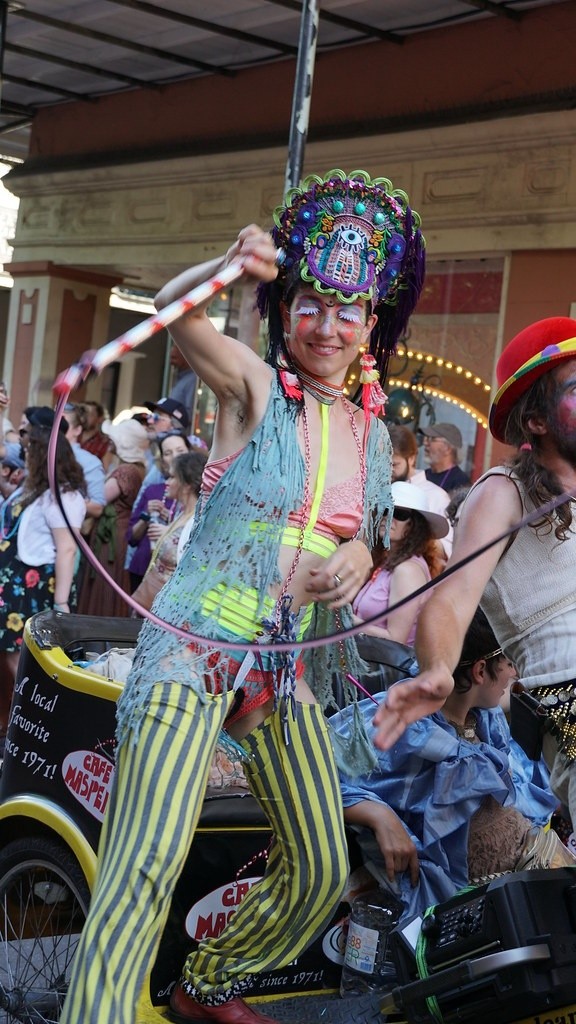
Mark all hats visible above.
[490,318,575,445]
[418,423,462,449]
[102,419,149,466]
[29,407,69,433]
[144,397,188,428]
[388,481,449,538]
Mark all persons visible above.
[0,358,457,670]
[321,607,557,924]
[60,169,428,1024]
[367,314,576,857]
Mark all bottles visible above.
[148,509,162,555]
[338,888,411,998]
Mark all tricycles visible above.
[0,612,576,1024]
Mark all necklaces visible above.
[280,357,345,406]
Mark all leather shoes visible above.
[169,979,280,1023]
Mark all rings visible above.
[333,574,341,587]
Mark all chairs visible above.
[37,612,420,887]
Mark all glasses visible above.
[66,403,75,412]
[426,436,442,442]
[381,507,413,522]
[19,429,30,436]
[157,429,182,438]
[147,414,172,421]
